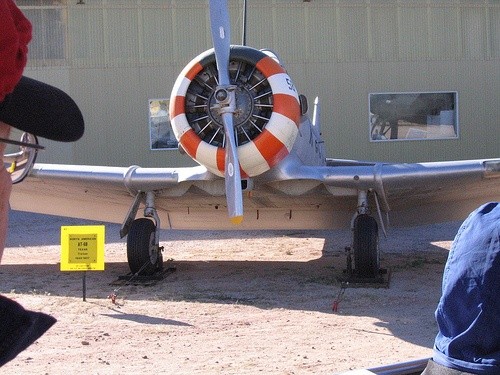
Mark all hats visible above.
[0,1,85,142]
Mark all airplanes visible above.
[5,0,500,275]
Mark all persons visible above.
[420,200,500,375]
[0,0,85,367]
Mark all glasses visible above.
[0,132,46,184]
